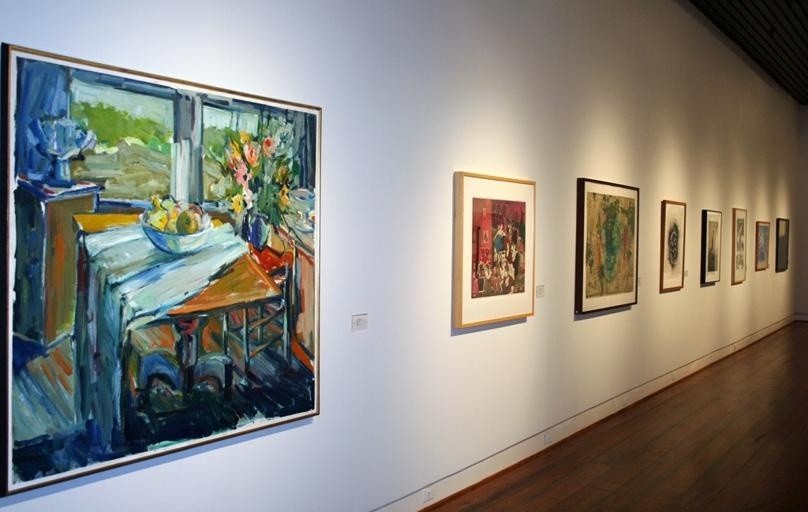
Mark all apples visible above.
[176,211,203,235]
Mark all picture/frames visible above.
[1,43,324,496]
[701,210,722,284]
[575,177,640,315]
[732,208,748,284]
[451,171,537,330]
[755,221,770,272]
[659,200,687,292]
[775,218,789,272]
